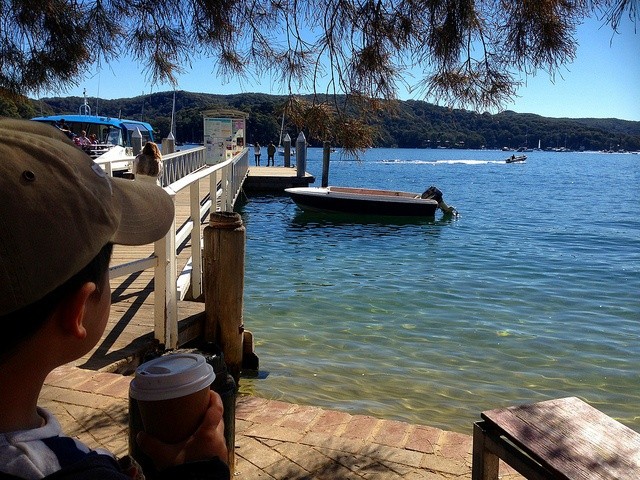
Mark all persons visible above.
[512,153,515,160]
[132,141,164,187]
[0,118,229,480]
[70,130,97,150]
[265,141,276,167]
[253,142,261,167]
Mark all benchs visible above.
[471,397,640,479]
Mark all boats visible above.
[284,186,439,222]
[505,155,527,163]
[278,145,296,156]
[29,88,155,174]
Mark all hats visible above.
[0,117,175,318]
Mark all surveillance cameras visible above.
[82,87,87,95]
